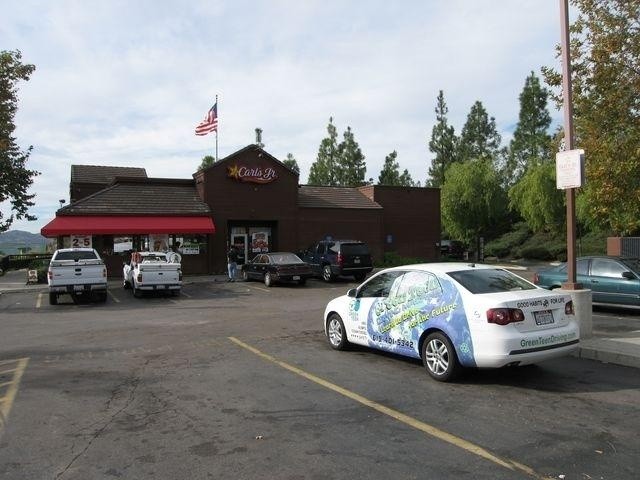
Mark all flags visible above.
[195,102,218,136]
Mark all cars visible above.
[534,255,639,306]
[122,253,183,297]
[323,261,579,382]
[241,240,373,286]
[441,239,463,255]
[47,247,107,305]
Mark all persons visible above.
[226,245,239,282]
